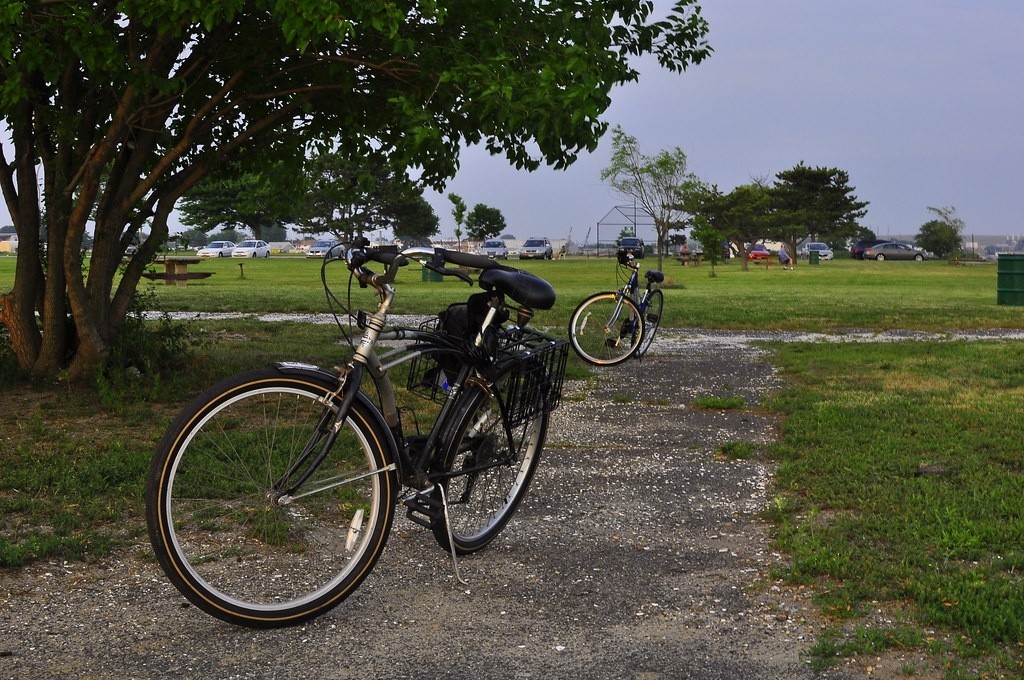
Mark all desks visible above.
[156,256,206,287]
[695,253,704,266]
[683,253,691,267]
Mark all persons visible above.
[779,245,793,270]
[679,242,690,265]
[558,246,566,256]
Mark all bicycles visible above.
[143,236,573,630]
[567,249,664,368]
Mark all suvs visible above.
[519,236,553,260]
[479,238,509,261]
[617,237,645,260]
[849,240,891,261]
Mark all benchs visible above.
[676,258,704,267]
[141,272,217,280]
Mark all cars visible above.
[861,242,930,262]
[305,240,347,260]
[801,242,834,262]
[197,241,237,259]
[231,239,271,259]
[744,243,772,261]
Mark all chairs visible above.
[779,256,791,270]
